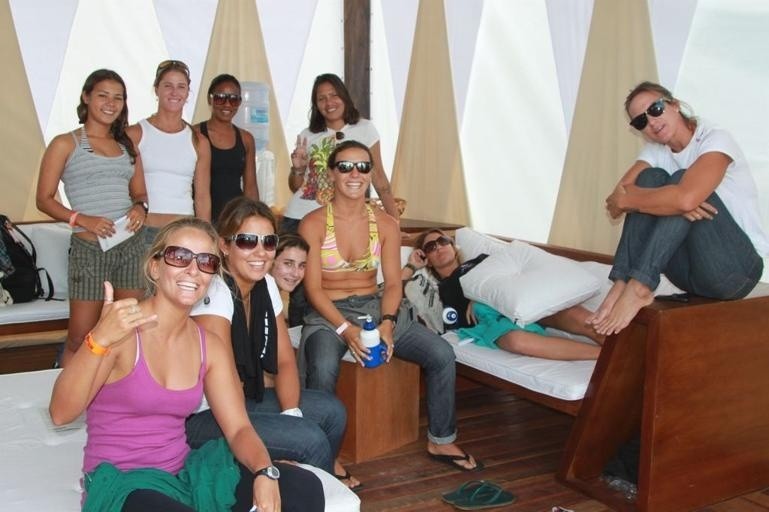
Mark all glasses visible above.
[212,93,242,107]
[424,235,449,253]
[153,247,220,274]
[334,161,372,174]
[234,233,279,252]
[159,61,190,75]
[629,97,671,130]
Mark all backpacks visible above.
[0,213,65,302]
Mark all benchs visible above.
[388,224,769,511]
[0,219,79,352]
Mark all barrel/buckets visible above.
[230,81,271,151]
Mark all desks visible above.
[332,358,422,463]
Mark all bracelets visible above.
[83,332,108,356]
[405,263,416,275]
[69,211,80,228]
[291,171,304,176]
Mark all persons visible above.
[401,228,608,361]
[48,218,325,512]
[276,73,400,235]
[583,82,768,337]
[268,231,311,293]
[191,73,259,227]
[291,140,484,491]
[187,197,350,475]
[123,59,212,300]
[35,69,149,370]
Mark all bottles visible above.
[357,318,389,369]
[442,304,460,326]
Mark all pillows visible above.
[458,239,602,327]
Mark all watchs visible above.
[252,466,280,480]
[382,315,398,327]
[133,201,148,215]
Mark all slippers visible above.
[454,487,516,511]
[443,478,499,503]
[429,445,484,473]
[333,467,364,492]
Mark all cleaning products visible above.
[357,313,387,369]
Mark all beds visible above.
[1,363,365,512]
